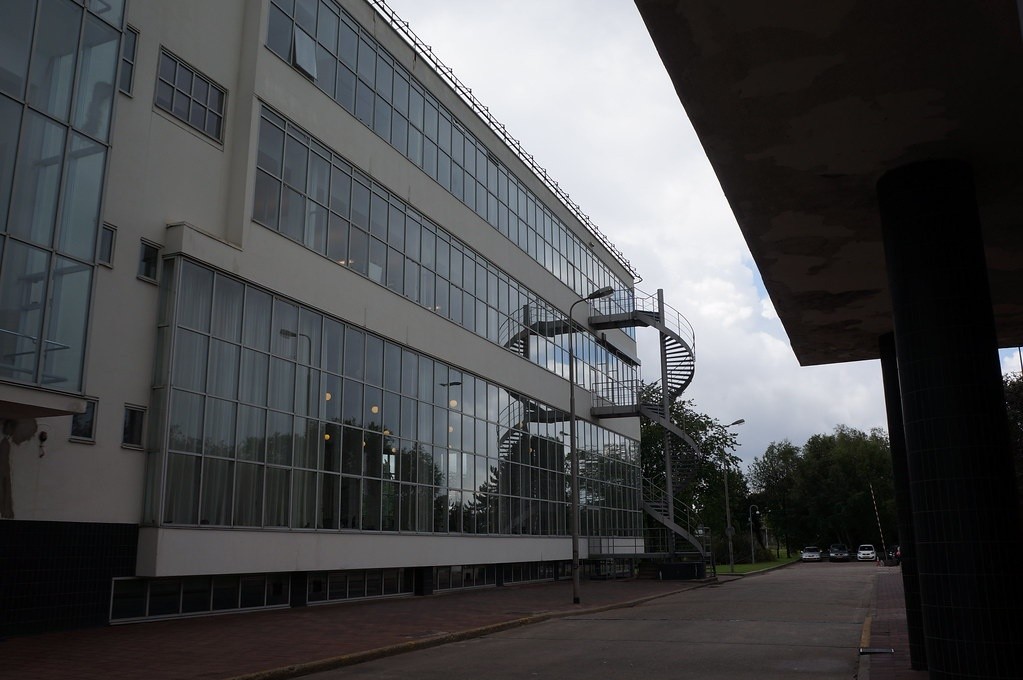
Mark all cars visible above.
[855,544,878,562]
[800,546,823,562]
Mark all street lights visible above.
[279,328,312,525]
[722,419,745,573]
[567,286,616,605]
[749,504,761,564]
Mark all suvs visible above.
[827,544,852,562]
